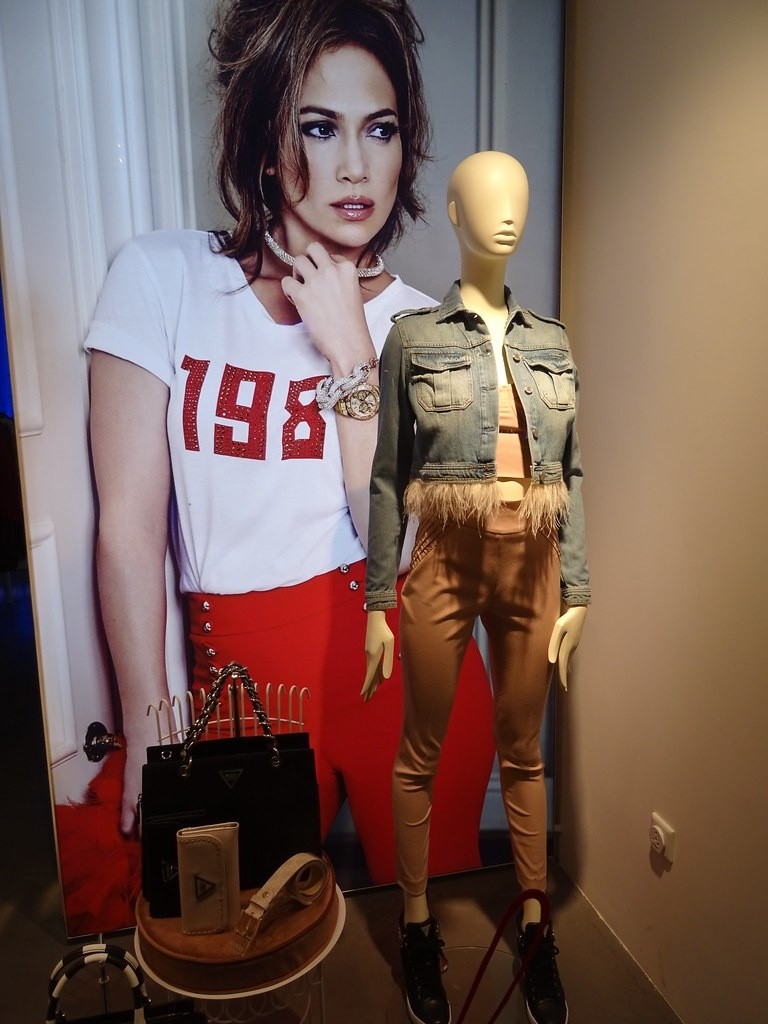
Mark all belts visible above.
[235,853,335,962]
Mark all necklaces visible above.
[260,228,384,278]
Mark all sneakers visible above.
[514,913,569,1024]
[396,913,455,1024]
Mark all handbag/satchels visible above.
[43,944,208,1024]
[139,663,323,920]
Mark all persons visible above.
[357,151,592,1024]
[82,0,501,937]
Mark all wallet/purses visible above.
[174,823,241,935]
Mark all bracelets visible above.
[316,357,382,409]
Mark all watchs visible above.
[334,382,381,422]
